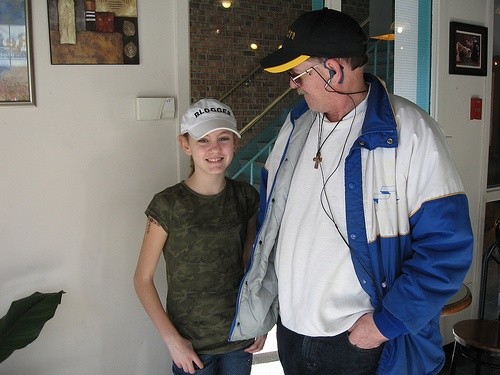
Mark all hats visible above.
[180,98,241,140]
[259,7,367,73]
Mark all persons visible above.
[228,6,474,375]
[134,98,268,375]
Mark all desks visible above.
[440,283,472,317]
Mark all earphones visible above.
[329,70,336,78]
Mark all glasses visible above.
[287,58,344,88]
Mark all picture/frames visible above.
[449,21,488,76]
[0,0,36,106]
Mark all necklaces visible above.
[313,110,354,169]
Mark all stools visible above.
[449,320,500,375]
